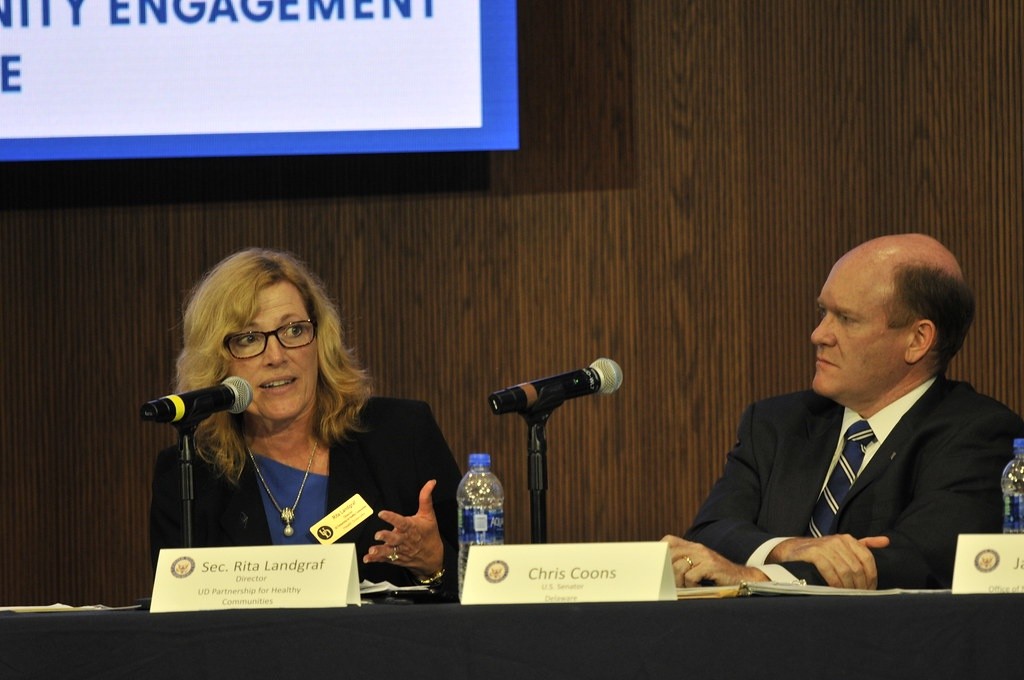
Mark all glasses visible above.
[223,319,319,360]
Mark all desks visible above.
[0,592,1024,680]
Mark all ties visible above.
[802,418,875,541]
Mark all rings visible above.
[387,547,399,562]
[683,556,693,566]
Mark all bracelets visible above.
[418,568,445,584]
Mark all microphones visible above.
[488,358,623,415]
[139,377,252,422]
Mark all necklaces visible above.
[242,428,319,537]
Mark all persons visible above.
[660,231,1024,589]
[149,247,463,601]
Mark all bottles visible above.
[457,453,505,604]
[1001,438,1024,534]
